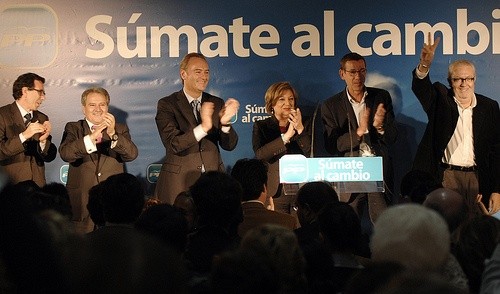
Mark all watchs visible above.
[110,134,118,141]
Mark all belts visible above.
[444,163,477,171]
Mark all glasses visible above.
[291,203,298,210]
[28,88,46,96]
[453,77,474,84]
[345,70,367,75]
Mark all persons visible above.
[411,33,500,216]
[252,82,314,216]
[58,87,138,235]
[154,52,239,207]
[0,158,500,294]
[320,53,395,228]
[0,73,57,187]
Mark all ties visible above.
[93,125,102,143]
[24,113,32,126]
[192,100,200,122]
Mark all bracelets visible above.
[220,124,230,128]
[420,64,429,68]
[281,133,290,143]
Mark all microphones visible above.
[346,112,353,156]
[310,112,317,158]
